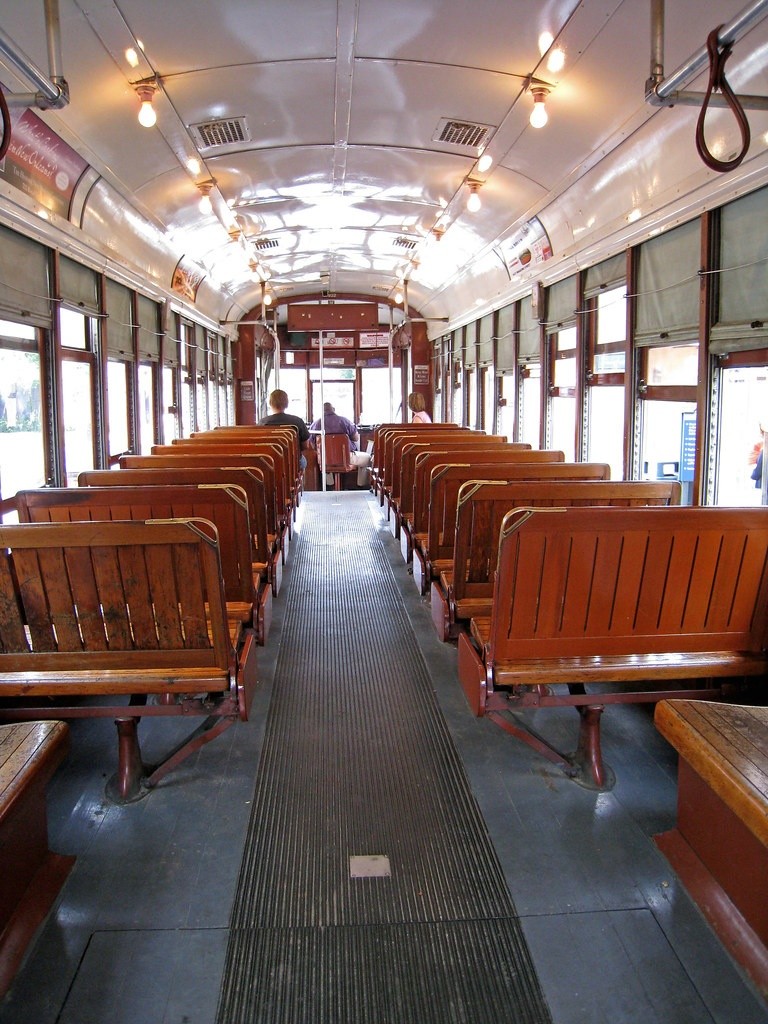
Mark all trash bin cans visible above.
[643,445,681,481]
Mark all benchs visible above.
[0,423,305,994]
[367,424,768,1007]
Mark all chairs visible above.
[316,434,358,491]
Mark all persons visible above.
[258,389,310,476]
[409,392,432,423]
[307,402,372,486]
